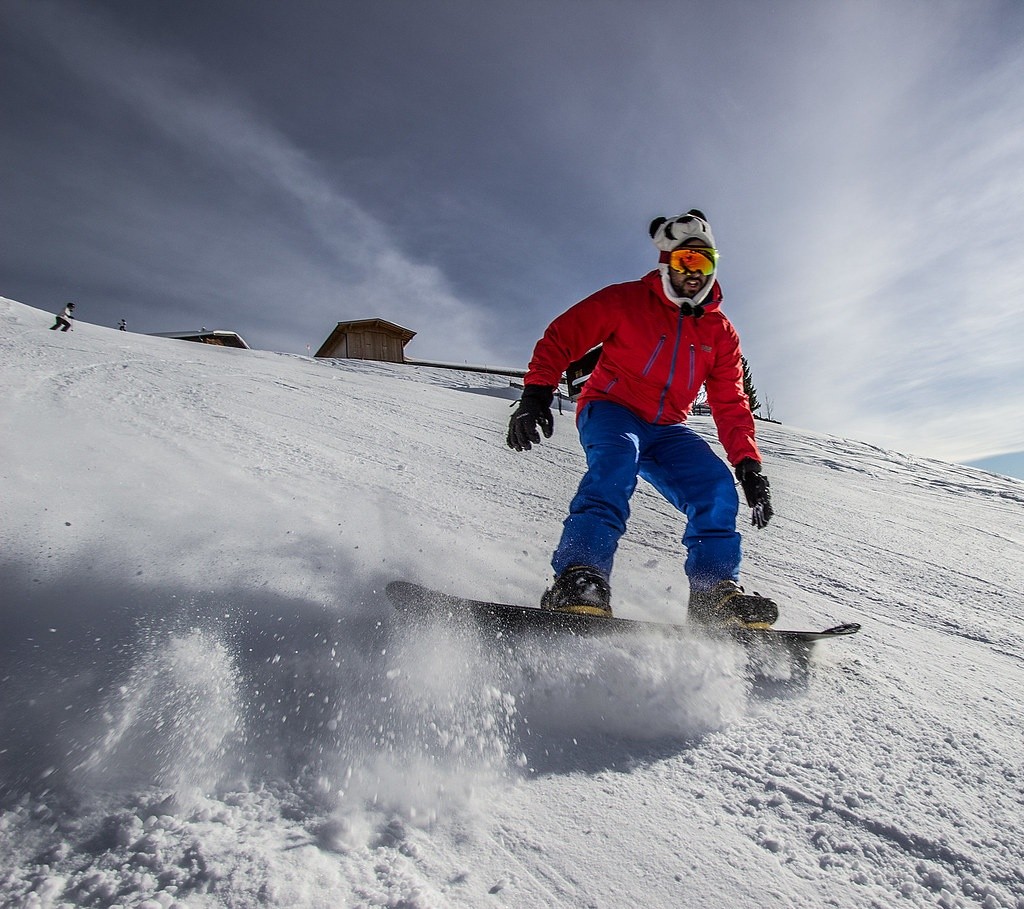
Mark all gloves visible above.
[118,322,120,323]
[123,322,126,324]
[735,456,774,529]
[68,315,75,318]
[506,384,563,452]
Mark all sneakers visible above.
[688,578,778,626]
[550,566,612,617]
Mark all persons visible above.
[50,303,75,332]
[506,208,787,630]
[117,318,127,332]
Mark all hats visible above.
[649,209,717,316]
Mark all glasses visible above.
[658,246,715,275]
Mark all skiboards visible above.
[383,577,865,646]
